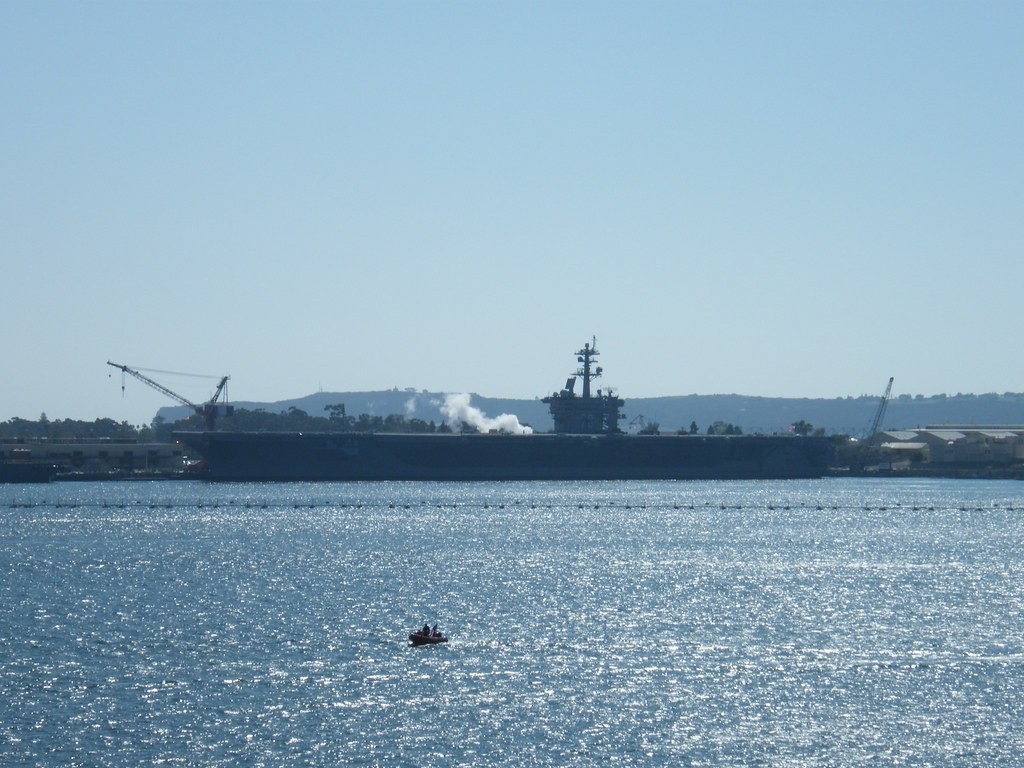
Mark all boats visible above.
[171,334,848,481]
[55,466,163,480]
[408,630,449,645]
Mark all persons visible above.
[417,623,442,637]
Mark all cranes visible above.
[849,375,894,469]
[105,360,233,429]
